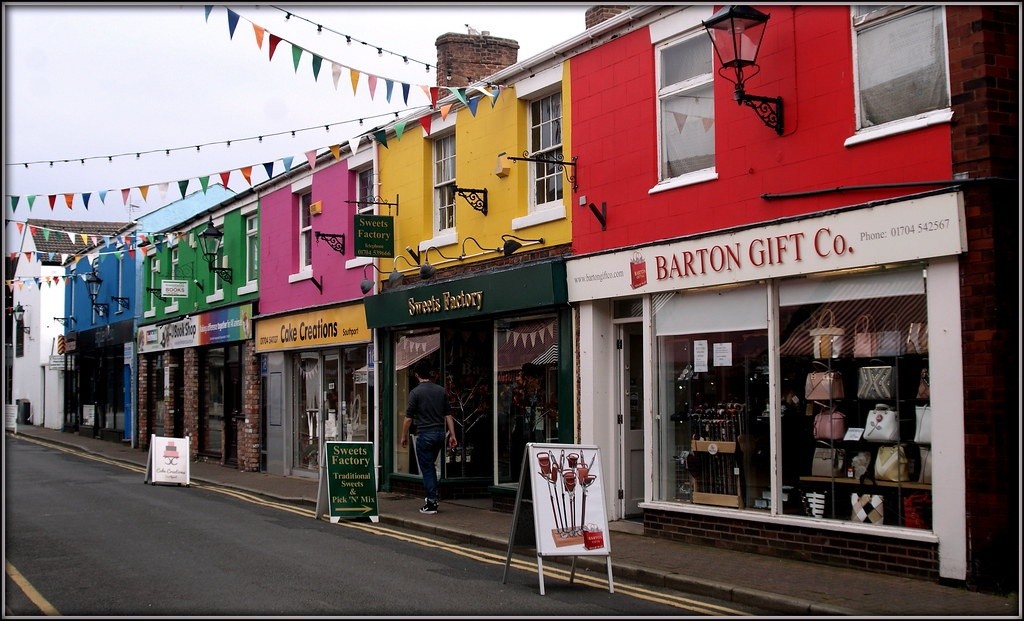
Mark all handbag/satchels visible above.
[853,315,877,357]
[905,322,929,353]
[851,471,883,526]
[863,403,899,442]
[803,488,824,518]
[857,360,895,399]
[875,313,902,356]
[914,405,932,443]
[806,361,844,400]
[811,440,844,478]
[903,493,932,529]
[810,309,845,359]
[875,438,907,482]
[918,447,933,484]
[917,369,930,399]
[851,451,876,479]
[814,402,845,439]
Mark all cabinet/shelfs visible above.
[799,354,933,489]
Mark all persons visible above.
[400,362,457,514]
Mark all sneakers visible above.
[419,499,438,514]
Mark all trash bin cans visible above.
[16,399,31,424]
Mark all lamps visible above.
[420,245,462,280]
[85,268,109,320]
[361,263,393,295]
[701,5,785,138]
[388,255,422,287]
[13,301,30,335]
[501,234,544,256]
[197,215,233,284]
